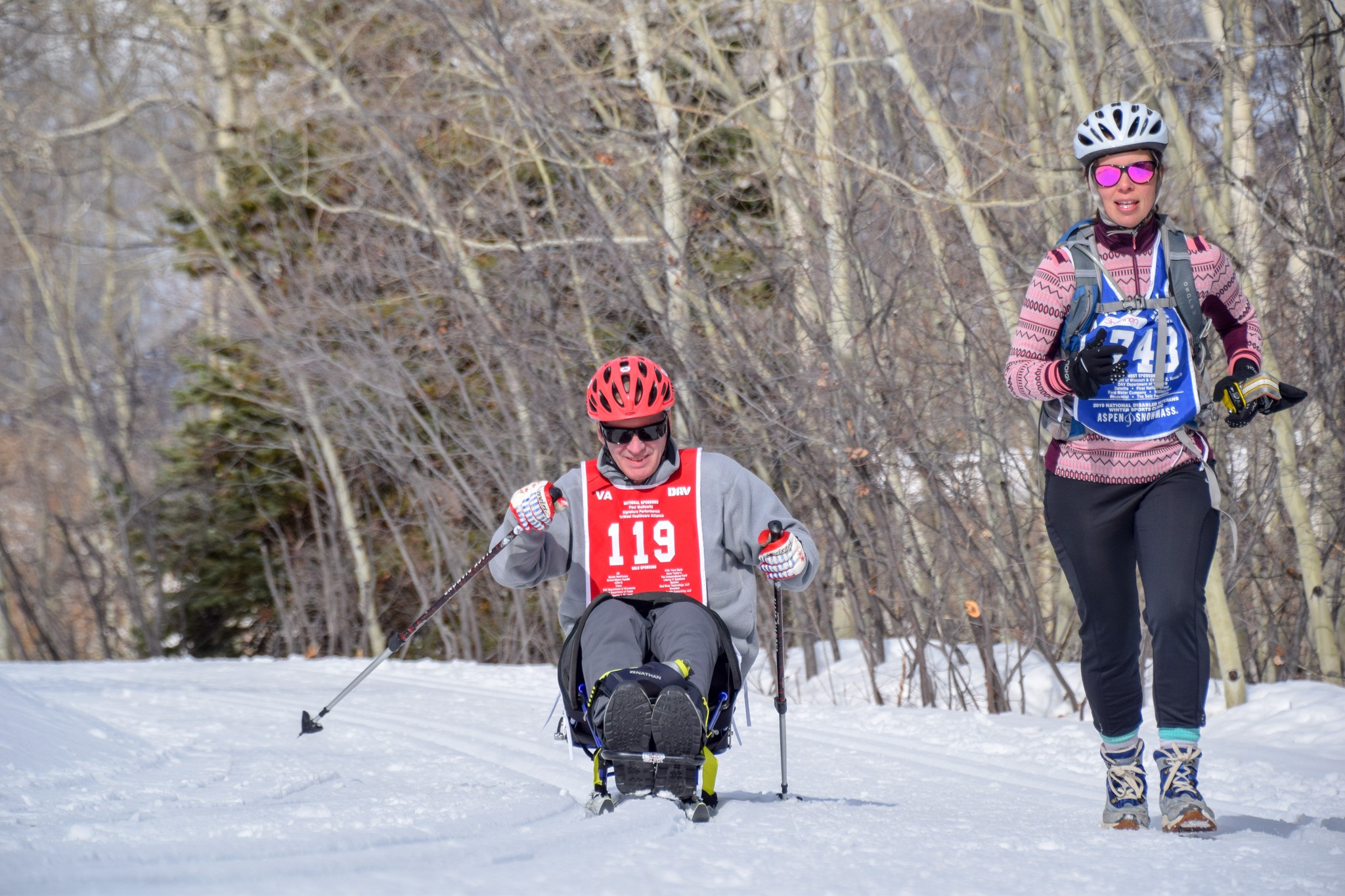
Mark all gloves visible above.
[1061,329,1128,399]
[509,480,569,534]
[1212,362,1259,429]
[758,529,806,579]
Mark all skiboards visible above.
[587,789,711,825]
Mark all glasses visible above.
[1093,162,1158,187]
[598,414,669,445]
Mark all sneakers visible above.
[1099,739,1149,831]
[1153,745,1216,832]
[650,686,703,803]
[602,680,650,797]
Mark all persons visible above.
[489,355,820,800]
[1003,101,1264,831]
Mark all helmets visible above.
[1074,102,1168,162]
[585,356,675,423]
